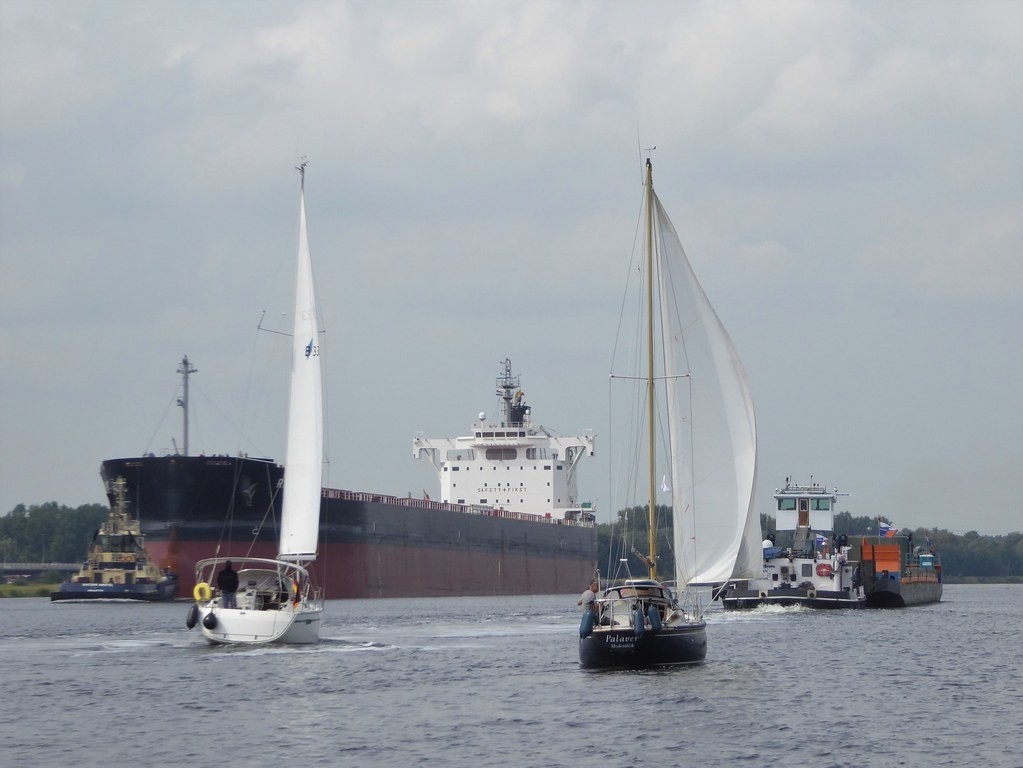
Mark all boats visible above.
[51,477,179,600]
[100,355,599,601]
[713,474,943,611]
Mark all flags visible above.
[879,521,898,538]
[927,538,936,552]
[292,577,302,608]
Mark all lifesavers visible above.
[192,582,212,602]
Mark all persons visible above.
[275,580,289,602]
[81,558,89,573]
[217,560,240,608]
[578,580,619,625]
[164,565,171,576]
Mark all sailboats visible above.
[190,157,324,644]
[577,124,758,673]
